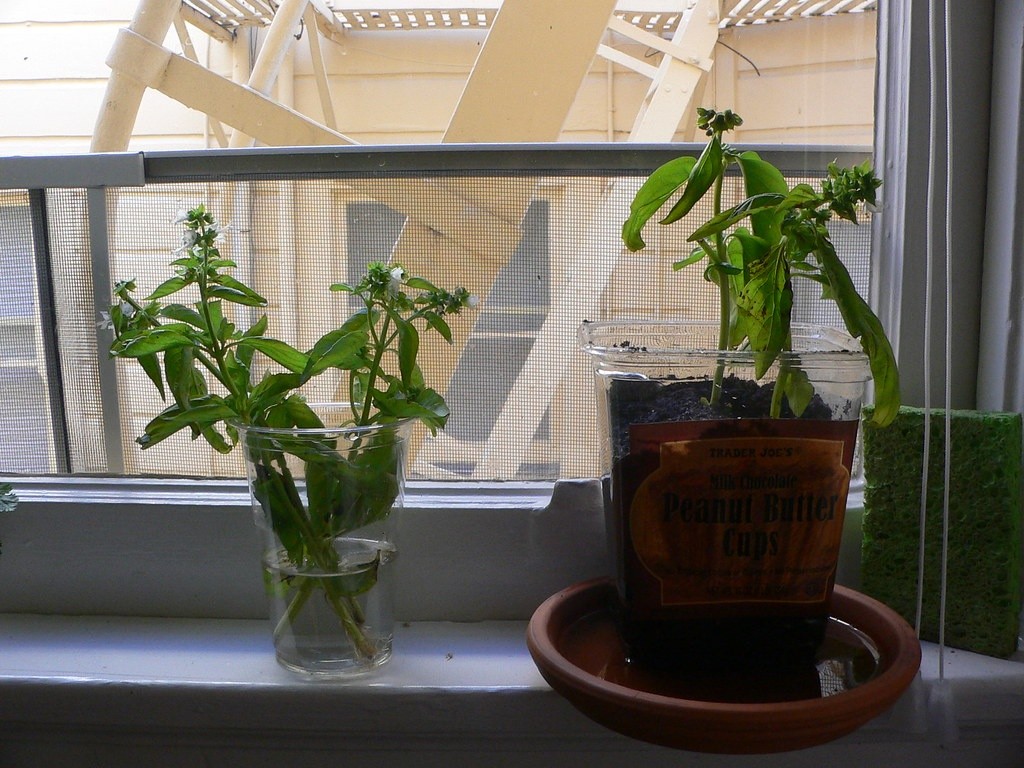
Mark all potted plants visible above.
[574,104,901,679]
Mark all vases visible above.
[222,403,420,677]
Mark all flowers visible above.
[93,204,483,660]
